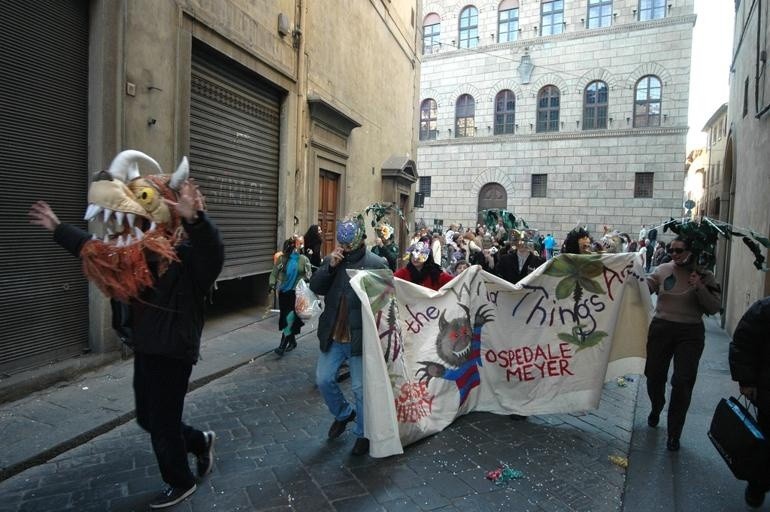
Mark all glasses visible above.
[668,247,685,255]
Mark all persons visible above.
[729,297,770,509]
[26,146,226,509]
[268,215,724,457]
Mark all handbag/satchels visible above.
[707,396,766,479]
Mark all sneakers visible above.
[352,439,369,455]
[150,484,197,507]
[667,436,680,452]
[745,481,765,507]
[328,408,356,438]
[197,432,214,478]
[648,409,660,426]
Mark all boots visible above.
[274,333,296,356]
